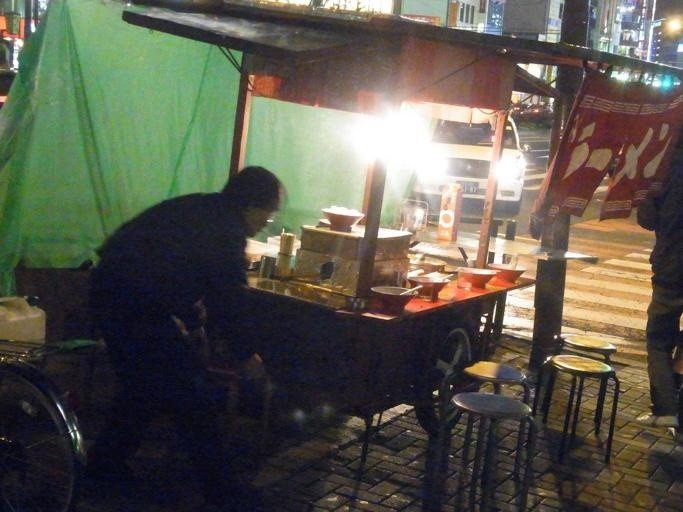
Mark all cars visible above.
[413,102,553,215]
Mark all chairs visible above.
[13,259,116,401]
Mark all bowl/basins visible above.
[458,263,527,288]
[371,277,450,313]
[321,208,365,233]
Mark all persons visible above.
[86,166,283,512]
[637,127,683,443]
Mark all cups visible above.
[258,255,276,279]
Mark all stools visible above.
[422,333,620,512]
[206,357,276,478]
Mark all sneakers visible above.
[636,411,683,442]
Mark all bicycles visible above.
[0,339,90,512]
